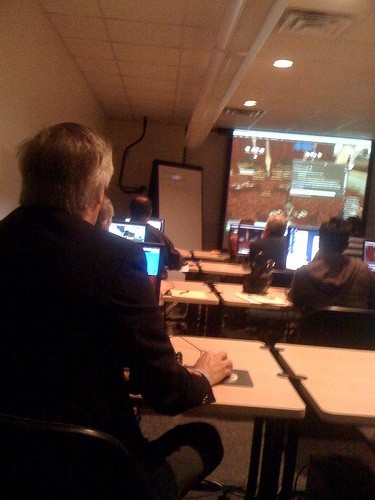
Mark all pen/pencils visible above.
[180,290,189,294]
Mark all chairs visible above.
[287,304,375,352]
[0,413,224,500]
[271,269,295,289]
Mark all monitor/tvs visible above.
[134,242,165,277]
[108,220,149,242]
[238,225,266,257]
[283,226,324,271]
[125,217,165,233]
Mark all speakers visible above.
[147,160,202,253]
[363,240,375,274]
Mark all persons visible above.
[288,217,375,350]
[95,195,114,231]
[0,123,233,500]
[229,218,254,263]
[250,214,289,269]
[343,217,366,260]
[128,196,184,270]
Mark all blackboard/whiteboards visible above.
[155,158,205,251]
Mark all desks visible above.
[121,248,375,500]
